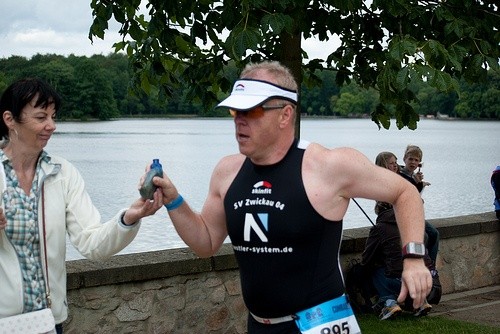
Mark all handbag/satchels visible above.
[344,259,374,314]
[427,267,442,304]
[0,308,57,334]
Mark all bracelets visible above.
[165,194,184,210]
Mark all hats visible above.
[214,79,298,110]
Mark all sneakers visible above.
[412,298,433,317]
[378,300,402,320]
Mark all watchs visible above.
[402,242,426,260]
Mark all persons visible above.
[0,77,166,333]
[137,61,433,333]
[490,166,500,221]
[362,145,443,321]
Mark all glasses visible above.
[229,104,295,119]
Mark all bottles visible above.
[151,159,162,169]
